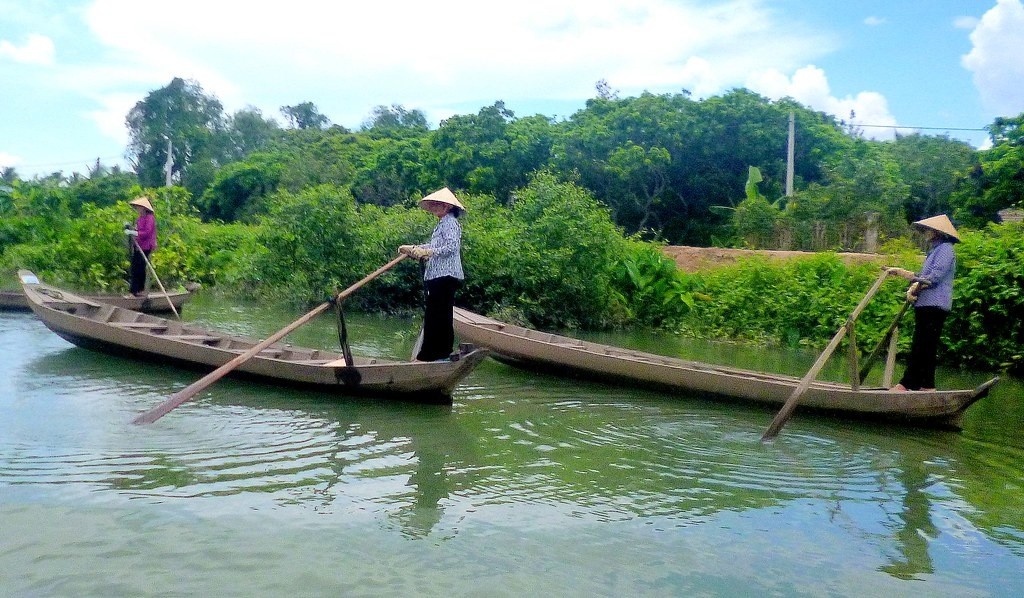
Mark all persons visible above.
[398,185,466,362]
[124,196,157,300]
[885,213,960,392]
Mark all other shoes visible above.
[919,387,936,392]
[123,293,135,300]
[889,383,906,392]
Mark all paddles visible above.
[758,268,889,441]
[124,223,181,321]
[134,248,418,423]
[855,276,932,385]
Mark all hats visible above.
[129,196,153,212]
[913,214,960,243]
[418,187,465,212]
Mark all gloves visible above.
[398,244,433,260]
[124,224,138,236]
[878,266,914,281]
[907,282,928,301]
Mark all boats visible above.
[18,268,490,427]
[0,223,190,313]
[451,265,1001,432]
